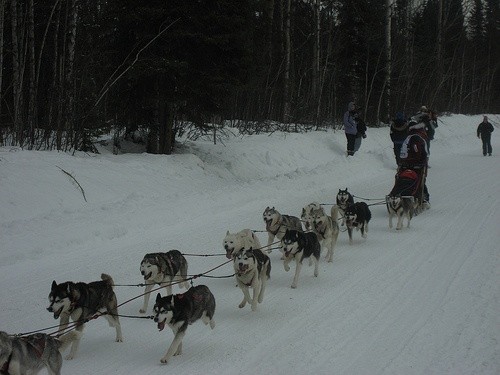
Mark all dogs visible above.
[153,285,216,364]
[387,192,418,230]
[223,229,272,312]
[46,273,124,360]
[0,330,81,375]
[263,201,339,289]
[139,249,190,314]
[337,188,372,245]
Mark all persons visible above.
[390,112,408,164]
[477,115,494,156]
[344,102,367,156]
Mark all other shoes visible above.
[426,201,430,210]
[484,154,491,156]
[347,155,359,160]
